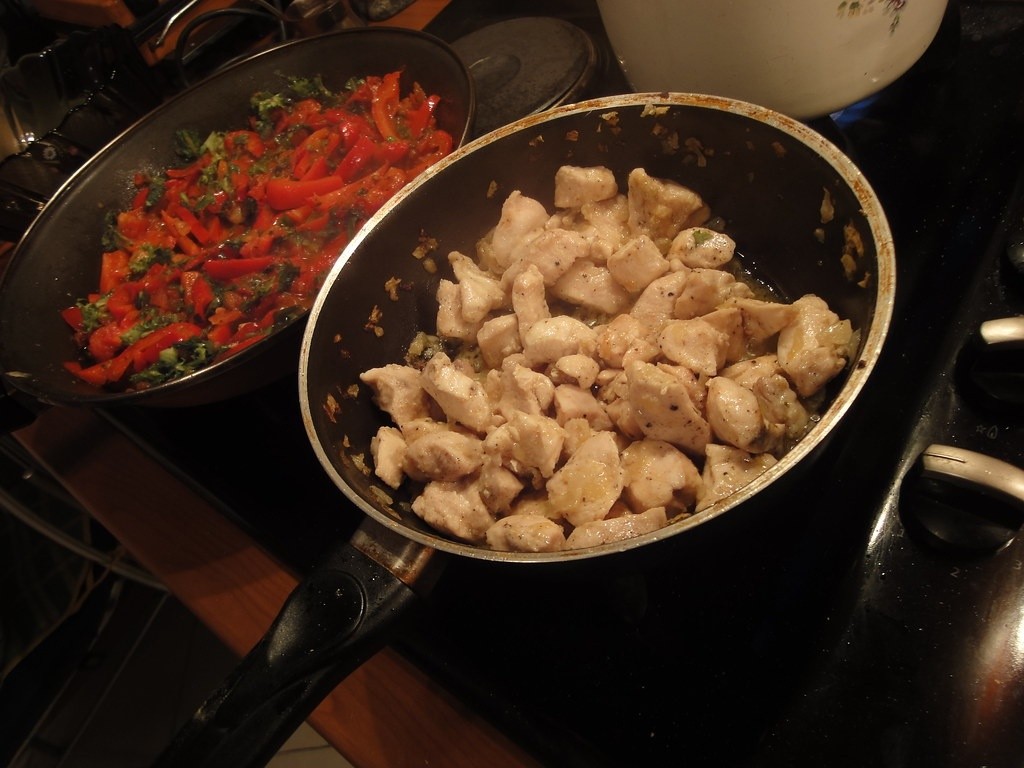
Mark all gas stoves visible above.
[88,1,1023,767]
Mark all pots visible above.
[594,1,947,120]
[1,12,476,414]
[147,87,897,767]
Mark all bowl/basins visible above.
[1,24,158,243]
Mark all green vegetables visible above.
[75,68,365,384]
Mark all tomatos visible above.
[63,74,452,378]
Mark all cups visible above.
[284,0,366,36]
